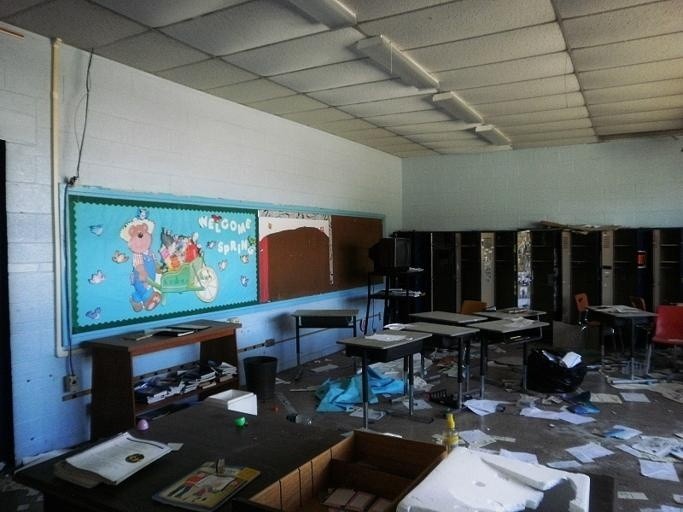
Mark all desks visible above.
[409,310,487,398]
[401,321,479,419]
[587,305,659,385]
[80,318,242,442]
[336,330,432,430]
[292,309,359,381]
[473,307,546,386]
[13,403,614,512]
[466,318,549,412]
[232,429,448,512]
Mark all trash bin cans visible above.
[243,356,277,400]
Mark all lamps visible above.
[357,33,439,91]
[290,1,358,31]
[433,91,484,123]
[476,124,512,146]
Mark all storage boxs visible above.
[206,389,257,416]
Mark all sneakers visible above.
[144,291,162,311]
[129,295,143,314]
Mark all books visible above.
[151,461,260,511]
[62,431,172,486]
[122,333,152,341]
[53,460,98,489]
[152,329,194,336]
[135,359,239,406]
[164,323,210,331]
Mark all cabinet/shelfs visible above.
[651,305,683,381]
[391,228,683,325]
[364,272,422,333]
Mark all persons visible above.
[166,470,205,499]
[181,485,212,502]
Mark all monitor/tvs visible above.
[368,237,415,273]
